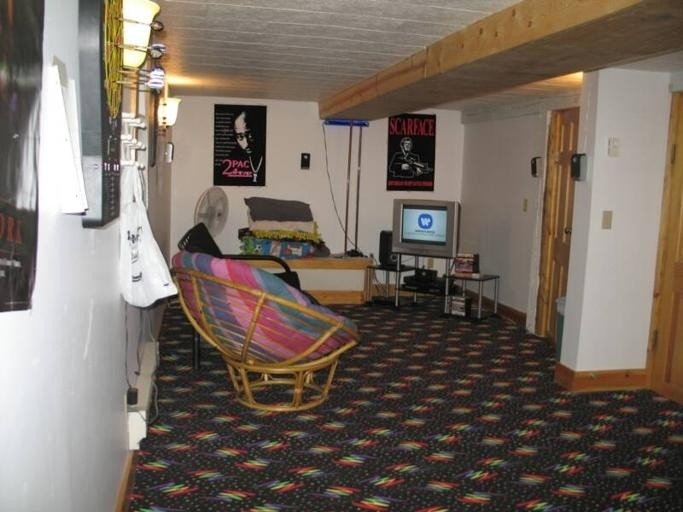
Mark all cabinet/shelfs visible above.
[366,252,499,319]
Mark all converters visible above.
[127,388,137,404]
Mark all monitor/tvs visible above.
[392,199,461,259]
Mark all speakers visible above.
[379,231,401,267]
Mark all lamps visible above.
[157,96,181,133]
[123,0,160,67]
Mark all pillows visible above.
[241,195,321,242]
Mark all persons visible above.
[389,137,424,185]
[221,111,266,187]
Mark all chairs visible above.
[176,222,321,370]
[169,251,361,411]
